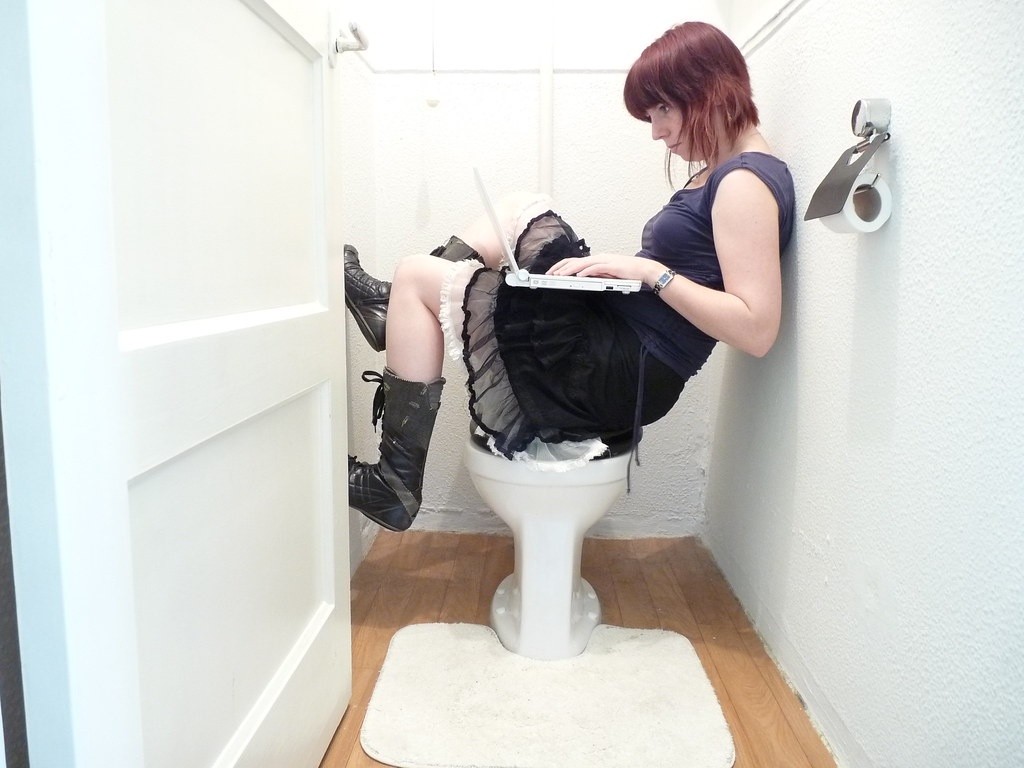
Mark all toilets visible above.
[464,413,645,661]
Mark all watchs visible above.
[653,270,676,294]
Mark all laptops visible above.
[474,166,641,293]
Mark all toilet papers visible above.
[816,174,893,233]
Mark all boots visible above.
[348,365,446,532]
[344,235,486,352]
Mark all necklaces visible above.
[692,176,701,184]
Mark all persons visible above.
[344,21,795,532]
